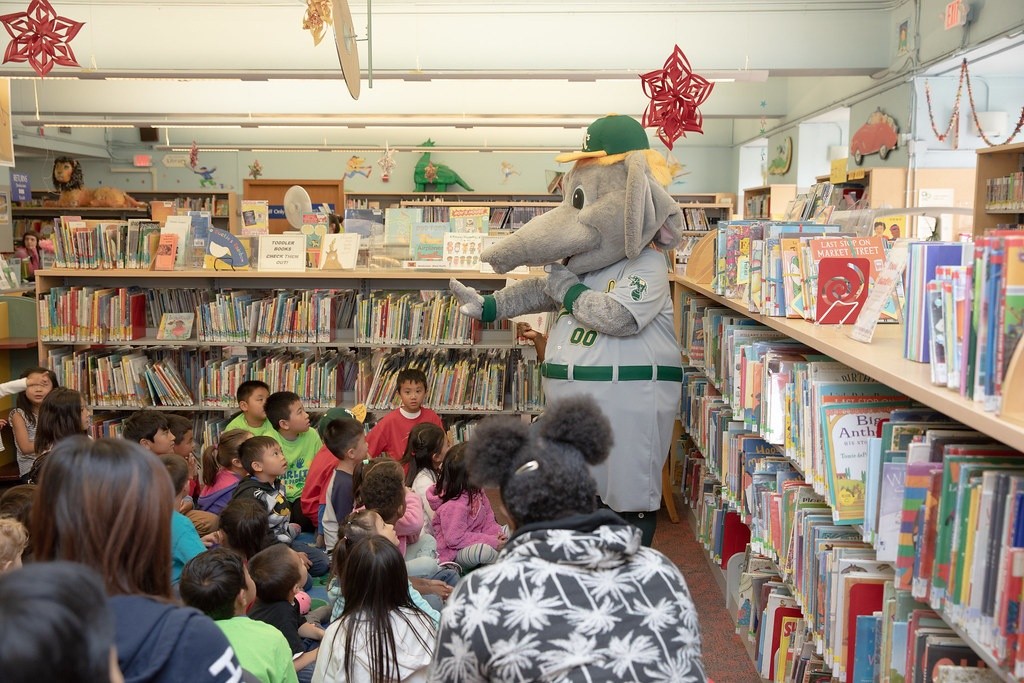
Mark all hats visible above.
[318,403,367,442]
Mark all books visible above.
[664,170,1024,683]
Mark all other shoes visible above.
[438,562,463,574]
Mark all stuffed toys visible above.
[450,115,683,552]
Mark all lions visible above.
[42,155,148,209]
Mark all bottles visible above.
[21,256,31,279]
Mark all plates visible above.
[284,186,312,229]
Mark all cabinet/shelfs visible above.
[0,141,1024,683]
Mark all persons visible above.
[873,222,889,241]
[889,224,900,241]
[13,231,42,280]
[0,367,704,683]
[0,191,564,455]
[328,214,343,234]
